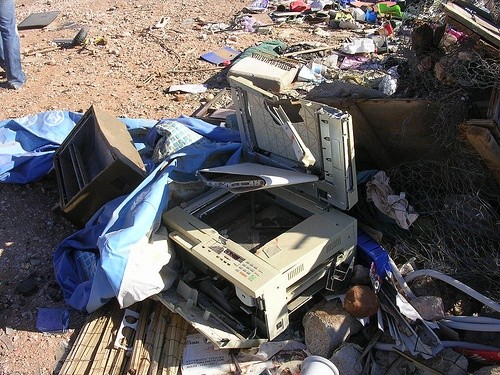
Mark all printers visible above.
[160,76,360,347]
